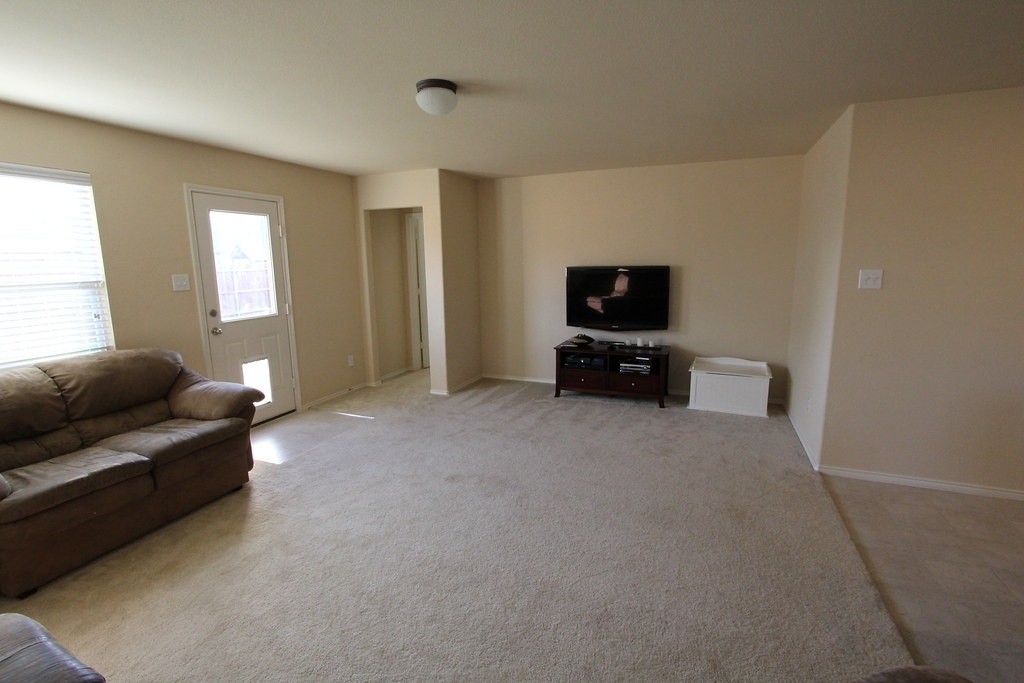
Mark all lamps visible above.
[415,78,459,117]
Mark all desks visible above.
[687,356,773,419]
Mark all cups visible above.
[649,339,655,347]
[637,337,644,346]
[625,339,631,346]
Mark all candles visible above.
[626,338,631,345]
[648,339,655,347]
[636,336,644,346]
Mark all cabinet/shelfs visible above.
[553,337,672,409]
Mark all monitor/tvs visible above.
[566,265,670,331]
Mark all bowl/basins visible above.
[570,334,593,345]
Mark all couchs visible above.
[0,612,107,683]
[0,347,265,599]
